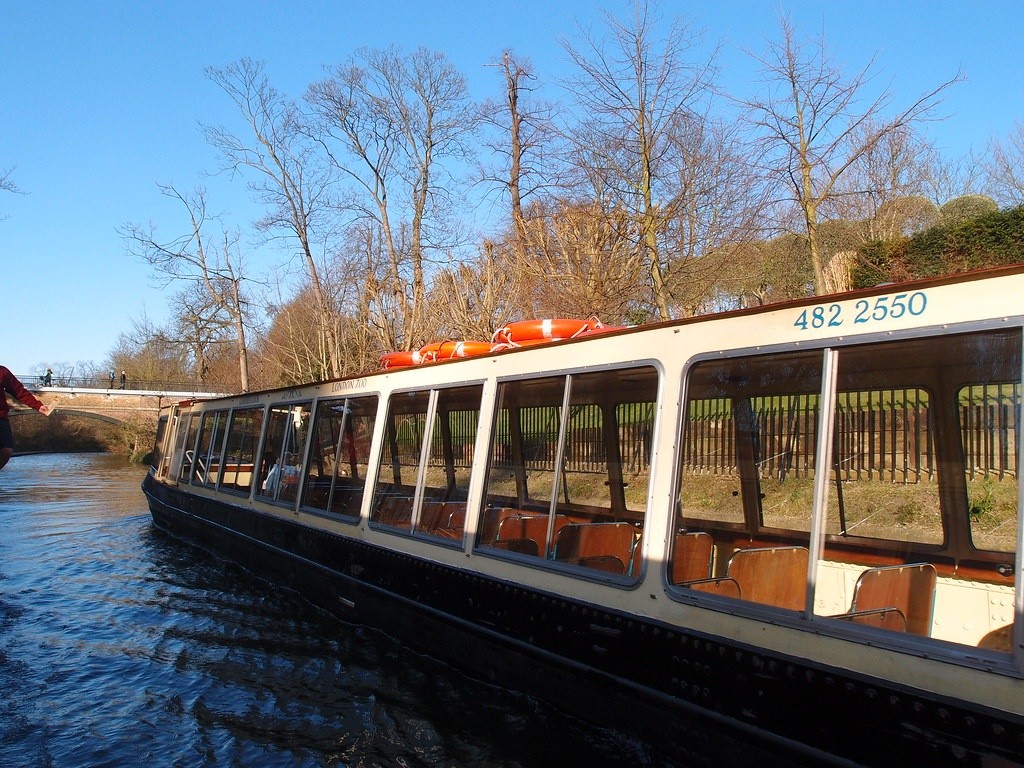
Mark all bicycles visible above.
[37,378,59,388]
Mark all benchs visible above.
[281,481,937,636]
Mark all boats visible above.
[142,267,1024,768]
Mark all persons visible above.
[118,370,127,390]
[109,370,115,389]
[265,446,301,497]
[44,368,53,387]
[0,366,48,470]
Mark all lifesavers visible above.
[493,318,637,354]
[419,340,499,358]
[378,351,427,367]
